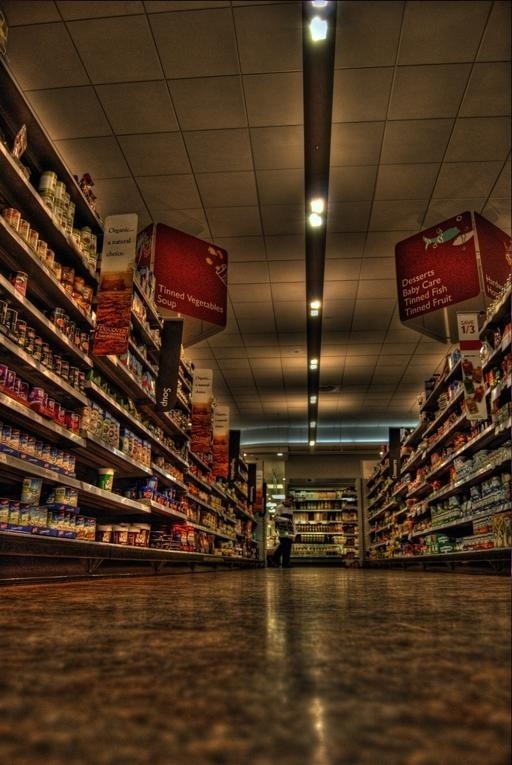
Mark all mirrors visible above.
[262,460,286,553]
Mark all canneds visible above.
[216,263,228,287]
[0,169,152,549]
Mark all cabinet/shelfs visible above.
[365,274,512,564]
[289,489,360,566]
[1,65,259,571]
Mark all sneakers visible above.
[282,559,293,568]
[267,558,281,568]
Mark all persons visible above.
[271,495,295,568]
